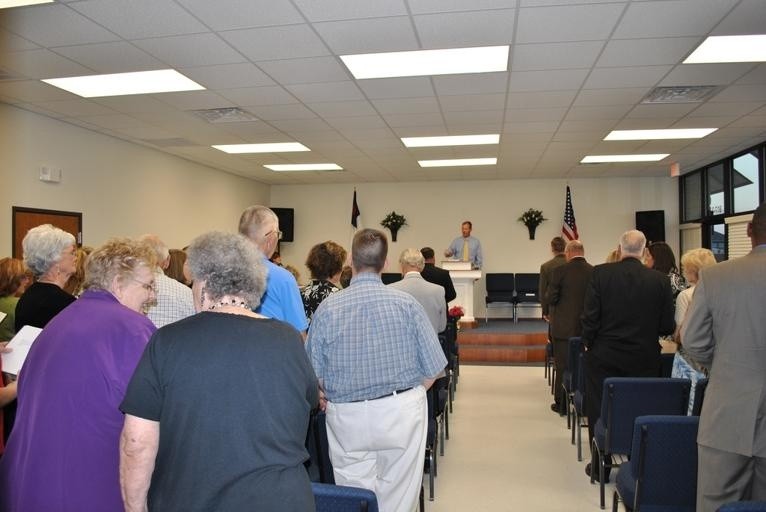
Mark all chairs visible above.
[308,323,459,512]
[544,333,765,511]
[484,272,541,323]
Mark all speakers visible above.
[268,207,295,242]
[635,210,665,247]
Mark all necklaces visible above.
[207,299,252,312]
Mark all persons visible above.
[538,234,719,416]
[1,205,455,487]
[680,201,766,512]
[117,231,319,512]
[540,238,595,415]
[579,228,676,484]
[304,229,449,511]
[445,221,482,272]
[0,237,161,511]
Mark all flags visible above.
[351,188,360,229]
[560,184,579,249]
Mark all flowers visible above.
[379,211,407,241]
[517,208,548,240]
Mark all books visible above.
[0,325,44,375]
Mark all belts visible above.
[352,387,413,402]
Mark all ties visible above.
[464,240,468,261]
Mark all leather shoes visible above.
[551,404,567,415]
[585,463,610,483]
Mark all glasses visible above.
[131,279,157,291]
[265,230,282,239]
